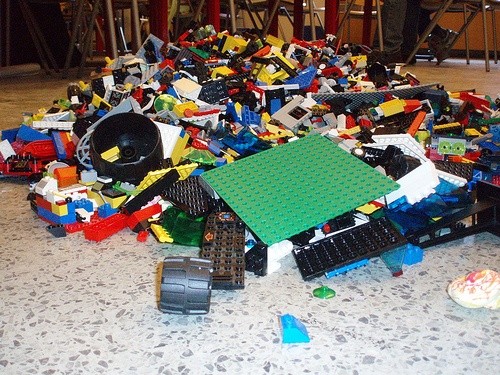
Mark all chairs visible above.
[0,0,500,72]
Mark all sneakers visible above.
[369,48,402,63]
[404,52,417,64]
[432,29,458,65]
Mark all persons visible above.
[366,0,420,66]
[27,0,82,68]
[420,10,459,59]
